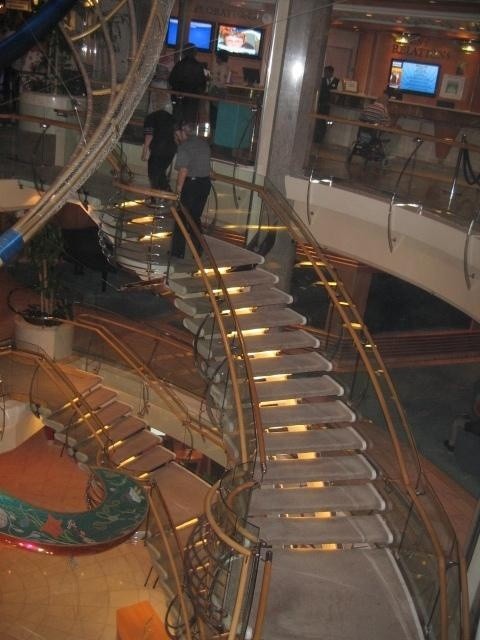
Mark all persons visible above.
[141,103,178,207]
[362,92,393,150]
[444,394,480,452]
[167,122,212,258]
[207,48,230,136]
[168,43,207,129]
[321,65,344,92]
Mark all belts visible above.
[187,177,200,181]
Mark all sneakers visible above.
[167,250,184,260]
[193,238,209,258]
[146,202,160,208]
[444,439,455,455]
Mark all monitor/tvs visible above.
[387,58,441,98]
[215,22,265,59]
[437,99,455,108]
[165,16,180,48]
[187,19,214,54]
[242,67,260,87]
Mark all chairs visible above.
[349,112,390,166]
